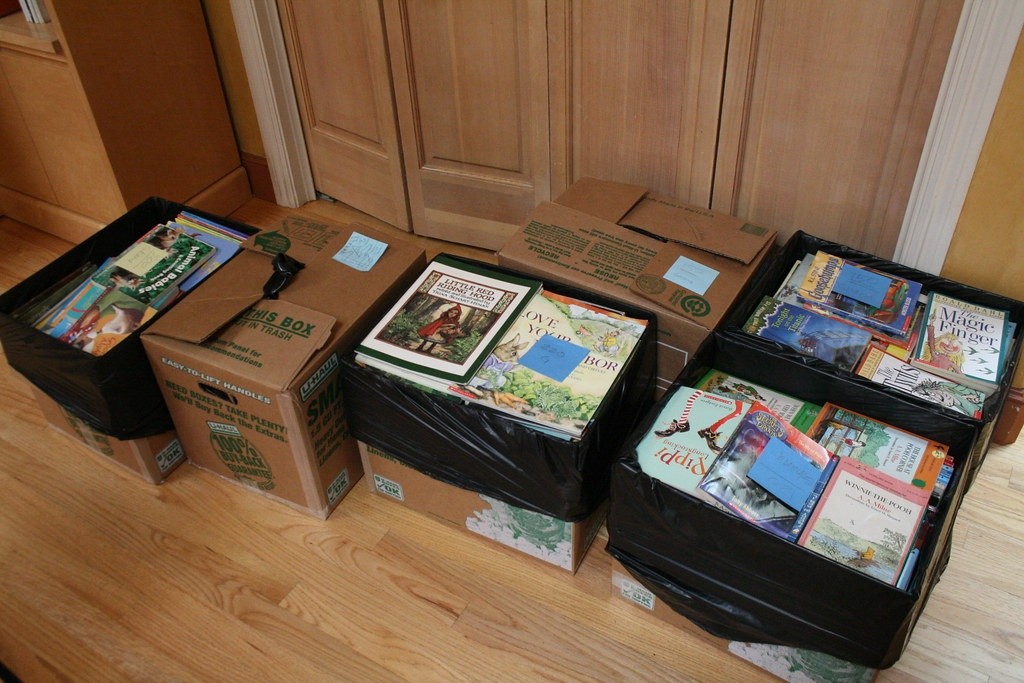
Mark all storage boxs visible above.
[713,231,1024,492]
[144,204,426,525]
[346,250,660,579]
[0,190,267,485]
[492,161,778,392]
[608,331,981,683]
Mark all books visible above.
[9,211,251,358]
[637,250,1019,593]
[18,0,50,24]
[351,252,647,445]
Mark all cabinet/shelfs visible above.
[0,0,254,244]
